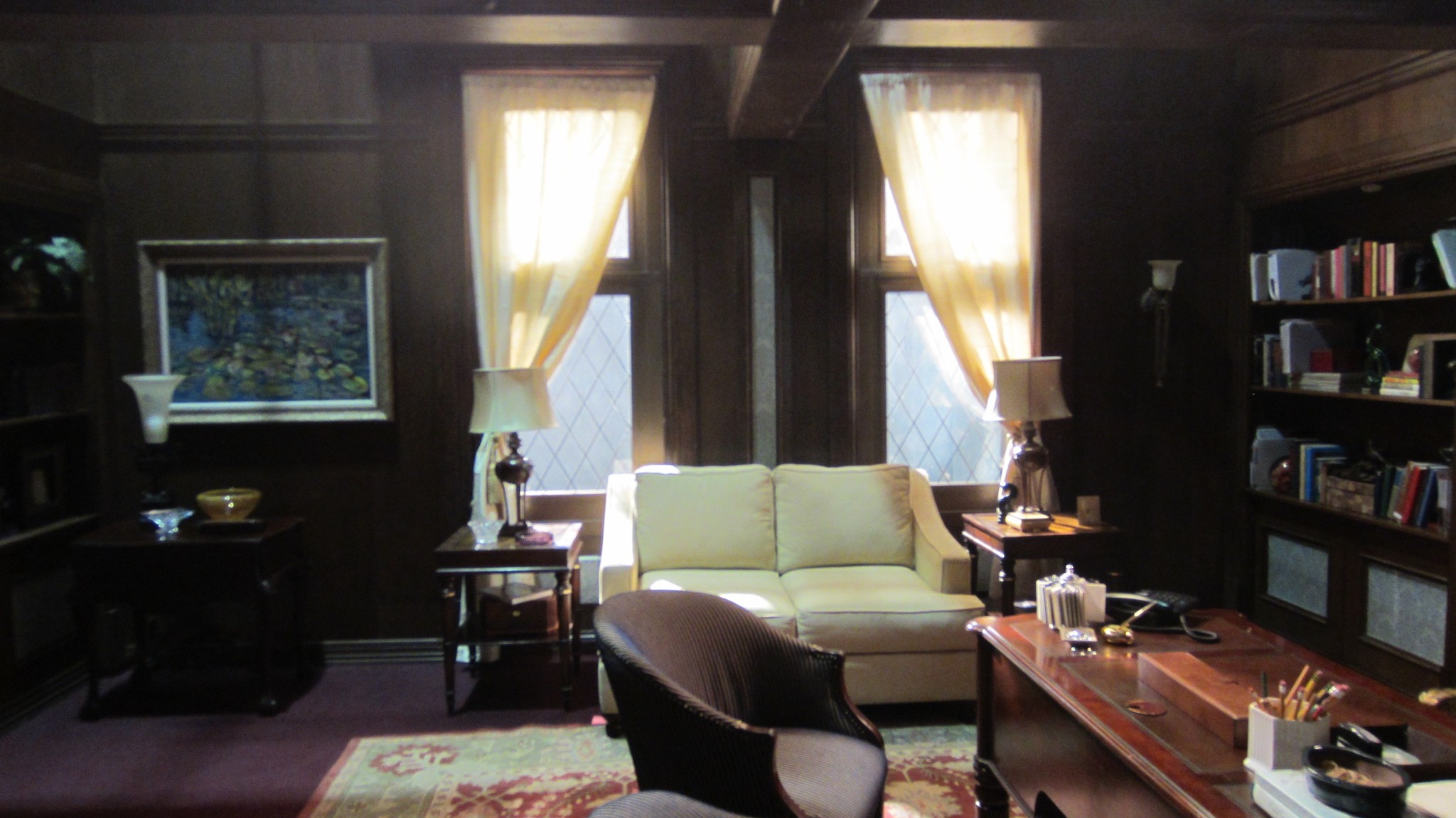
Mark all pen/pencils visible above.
[1250,688,1265,712]
[1278,680,1286,719]
[1260,670,1351,721]
[1284,664,1309,710]
[1120,600,1157,627]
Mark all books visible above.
[1301,370,1343,393]
[1379,371,1423,398]
[1376,461,1452,536]
[1299,442,1345,502]
[1312,239,1405,298]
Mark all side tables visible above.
[960,511,1113,616]
[433,519,585,713]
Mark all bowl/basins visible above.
[196,488,261,521]
[141,507,193,533]
[1302,745,1412,818]
[467,520,506,544]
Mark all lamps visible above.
[118,369,185,519]
[1135,257,1185,390]
[983,355,1074,520]
[467,363,562,537]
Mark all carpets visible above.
[300,722,1029,818]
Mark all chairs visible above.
[593,589,890,818]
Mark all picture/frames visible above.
[135,238,397,425]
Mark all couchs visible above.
[593,462,986,712]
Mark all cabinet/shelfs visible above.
[71,515,314,727]
[1255,288,1456,550]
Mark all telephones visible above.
[1105,588,1199,623]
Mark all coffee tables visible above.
[965,611,1456,818]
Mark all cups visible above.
[1246,696,1333,769]
[1077,496,1101,526]
[1267,249,1315,300]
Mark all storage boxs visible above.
[1327,471,1380,516]
